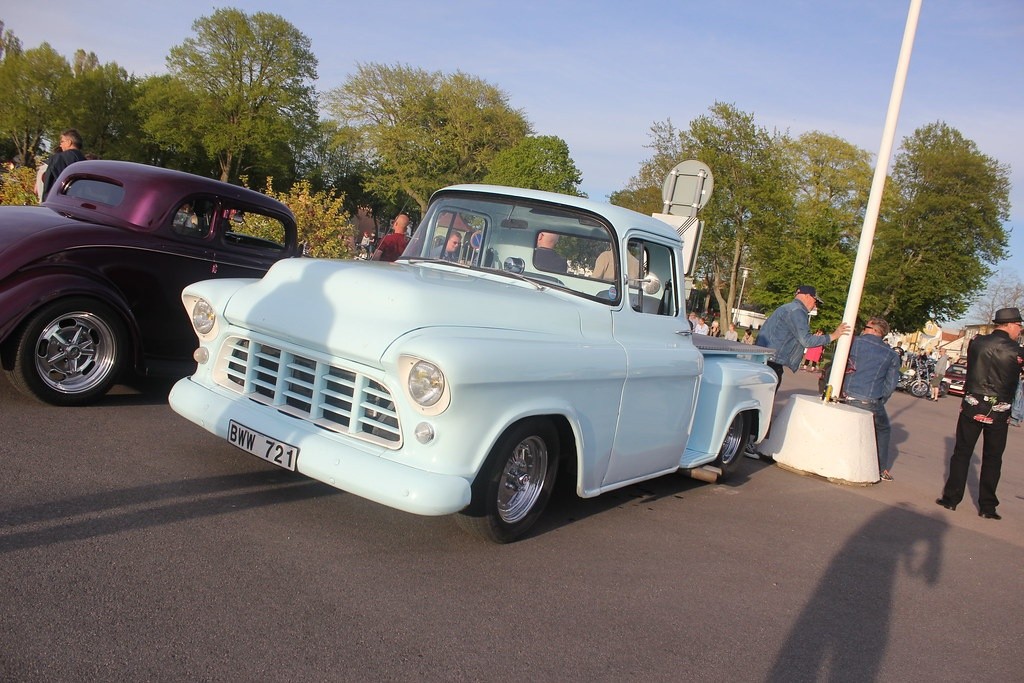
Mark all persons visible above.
[337,232,375,260]
[34,147,64,205]
[741,285,852,459]
[707,320,721,338]
[882,338,952,402]
[42,130,88,203]
[935,306,1024,520]
[373,214,412,262]
[687,312,709,335]
[800,327,825,372]
[741,329,754,345]
[429,231,463,262]
[724,322,738,341]
[590,242,647,288]
[534,231,567,274]
[839,316,900,482]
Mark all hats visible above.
[991,308,1024,323]
[797,286,823,305]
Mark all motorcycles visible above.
[896,351,933,398]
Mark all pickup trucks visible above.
[167,182,780,544]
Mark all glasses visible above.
[450,240,461,245]
[864,324,880,333]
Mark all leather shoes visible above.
[936,497,956,511]
[979,508,1002,520]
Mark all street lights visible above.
[732,267,753,324]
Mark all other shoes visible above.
[743,447,761,460]
[879,470,893,481]
[926,396,938,401]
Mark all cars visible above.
[1,161,309,405]
[939,357,971,396]
